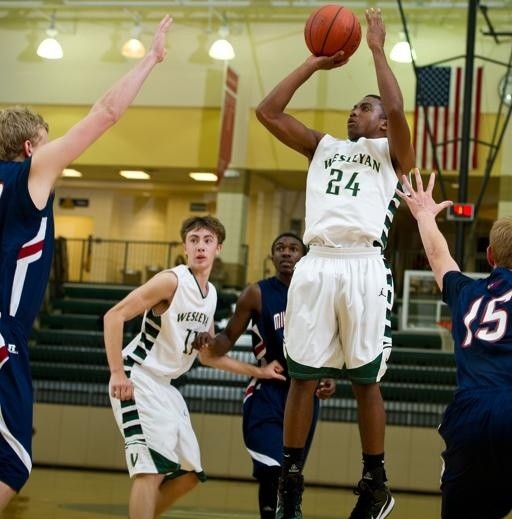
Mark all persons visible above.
[252,5,416,518]
[190,233,338,519]
[103,213,286,518]
[0,11,175,519]
[392,162,511,517]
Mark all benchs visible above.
[28,281,459,428]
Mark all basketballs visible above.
[305,5,361,63]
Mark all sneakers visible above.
[348,480,396,519]
[275,474,304,519]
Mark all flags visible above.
[411,63,481,171]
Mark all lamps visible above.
[209,10,236,60]
[390,14,416,63]
[35,7,64,59]
[122,9,151,58]
